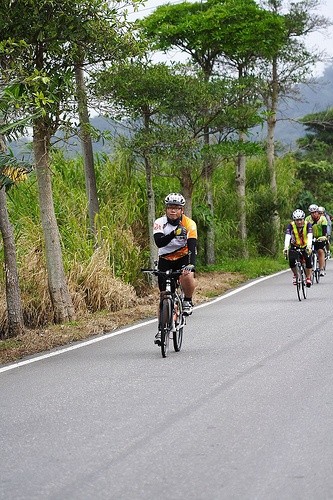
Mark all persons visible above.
[153,193,197,344]
[283,210,312,285]
[305,204,331,276]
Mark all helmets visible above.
[308,204,319,212]
[164,193,186,206]
[292,209,305,220]
[318,206,325,213]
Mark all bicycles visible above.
[140,267,193,357]
[284,238,331,301]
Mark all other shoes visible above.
[327,250,330,255]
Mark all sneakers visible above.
[182,300,193,315]
[306,278,311,287]
[292,274,297,285]
[154,330,163,344]
[319,269,324,276]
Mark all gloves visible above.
[282,248,288,255]
[306,248,312,254]
[317,236,327,241]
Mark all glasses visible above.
[167,206,182,212]
